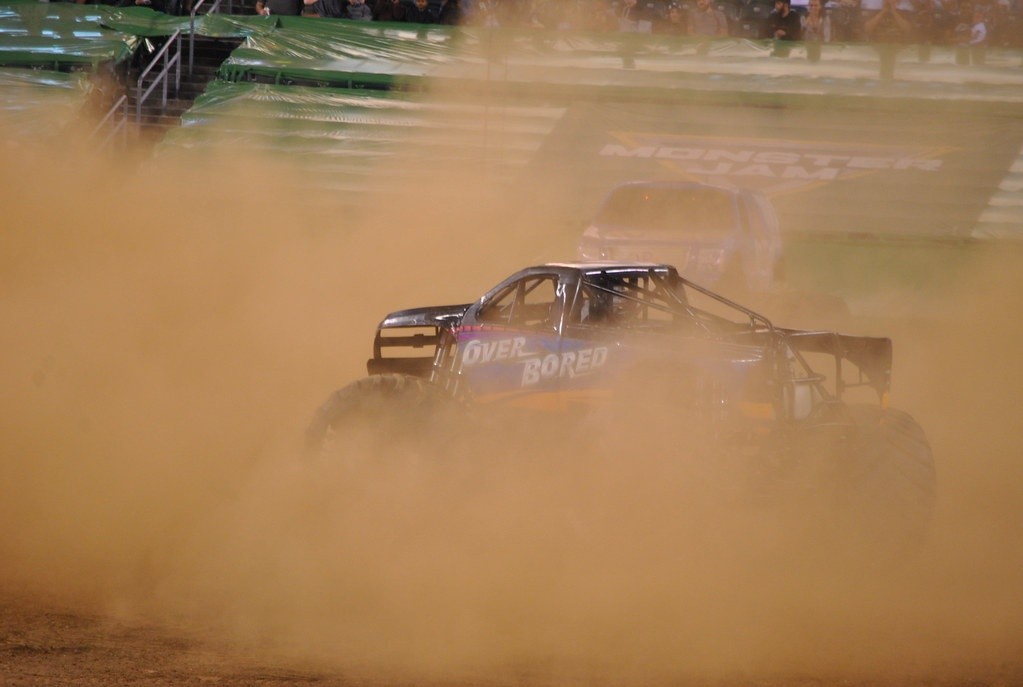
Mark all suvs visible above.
[576,178,790,295]
[302,260,937,507]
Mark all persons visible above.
[613,0,1023,42]
[67,0,186,16]
[254,0,459,26]
